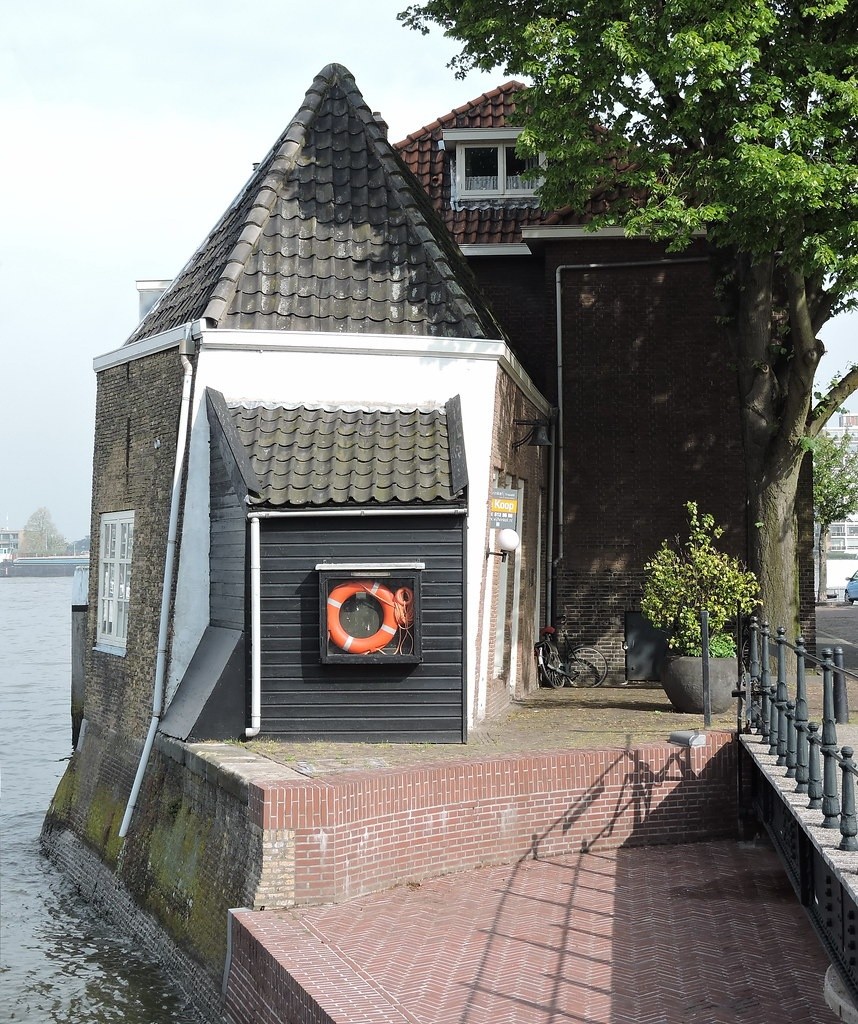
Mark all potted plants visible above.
[640,500,763,714]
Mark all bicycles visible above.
[534,615,608,690]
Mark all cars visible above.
[844,568,858,605]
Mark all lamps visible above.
[486,528,519,562]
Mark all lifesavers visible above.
[327,579,401,654]
